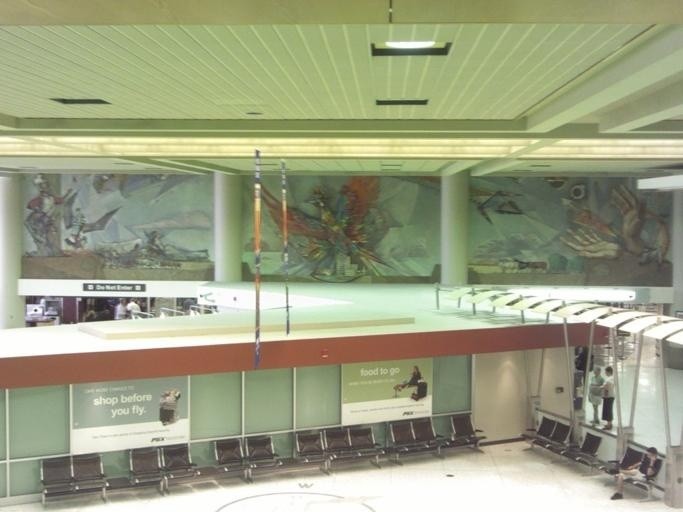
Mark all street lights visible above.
[589,383,602,395]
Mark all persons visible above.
[84,298,146,322]
[588,366,615,430]
[611,447,662,500]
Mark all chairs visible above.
[39,411,487,505]
[611,492,624,500]
[605,467,619,474]
[520,412,661,503]
[590,419,613,430]
